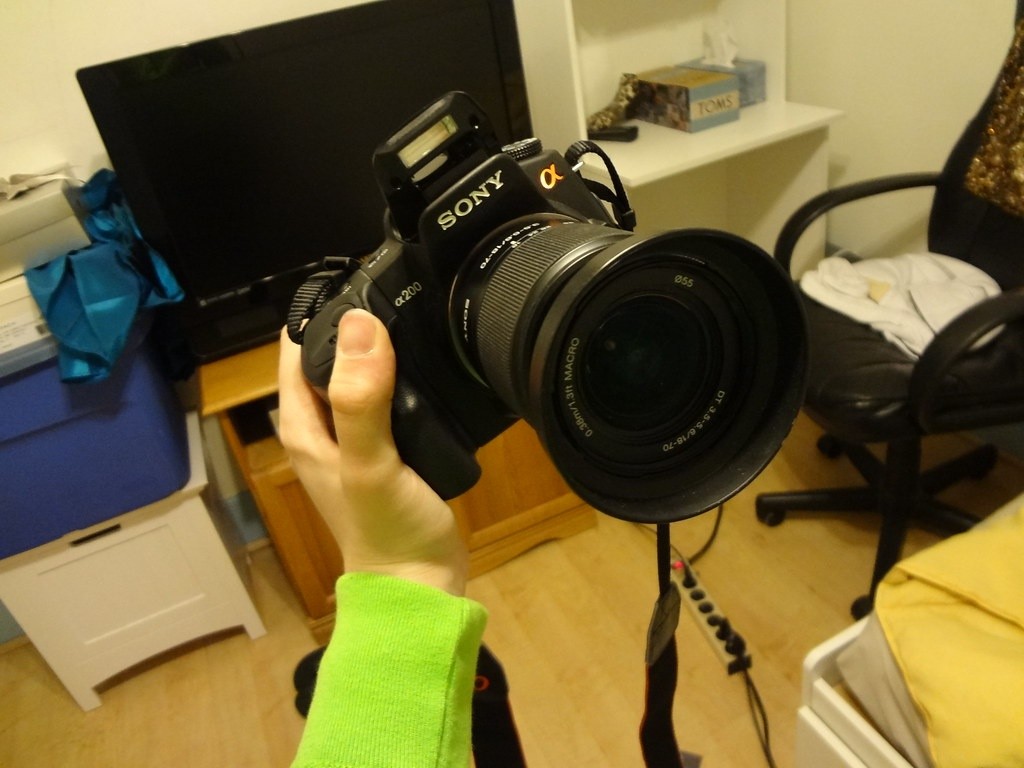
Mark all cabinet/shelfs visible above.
[511,1,839,283]
[199,341,599,652]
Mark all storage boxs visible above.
[1,275,59,378]
[0,355,191,562]
[625,64,742,134]
[0,160,90,285]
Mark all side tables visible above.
[1,411,267,711]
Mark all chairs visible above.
[754,2,1024,617]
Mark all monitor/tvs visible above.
[73,0,533,364]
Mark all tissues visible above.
[674,19,768,110]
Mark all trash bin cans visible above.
[293,642,529,768]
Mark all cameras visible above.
[299,90,808,525]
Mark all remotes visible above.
[586,125,638,140]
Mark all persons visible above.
[278,306,492,768]
